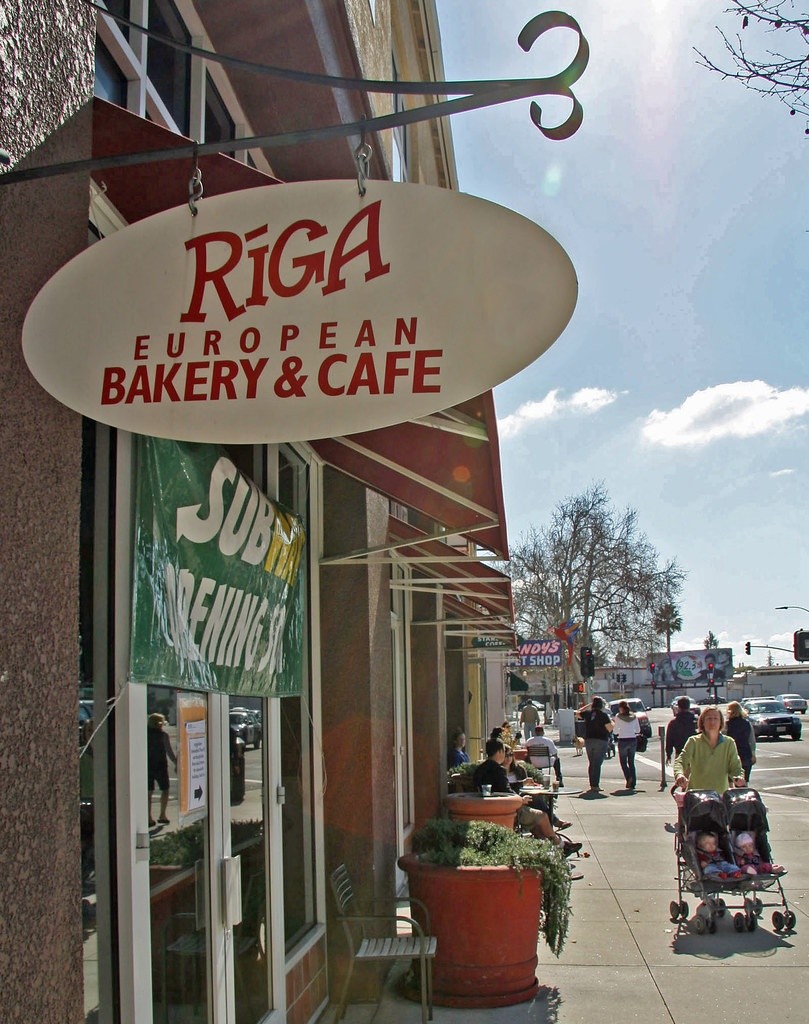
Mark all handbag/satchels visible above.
[636,733,648,752]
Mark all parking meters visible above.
[658,726,667,792]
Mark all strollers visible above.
[669,776,796,934]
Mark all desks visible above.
[445,789,522,831]
[519,785,582,857]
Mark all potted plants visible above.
[399,816,572,1008]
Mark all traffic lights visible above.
[578,683,583,692]
[622,674,627,682]
[708,662,714,669]
[650,662,655,673]
[616,674,620,682]
[709,679,714,684]
[580,646,594,677]
[745,642,750,655]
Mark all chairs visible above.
[328,863,438,1024]
[166,873,266,1018]
[527,746,557,781]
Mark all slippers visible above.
[555,821,573,832]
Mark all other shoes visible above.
[558,780,564,787]
[562,841,582,858]
[590,784,604,792]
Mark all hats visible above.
[735,833,753,847]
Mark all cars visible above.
[608,698,652,742]
[518,700,544,711]
[229,707,262,749]
[740,693,807,739]
[700,695,725,704]
[670,695,701,716]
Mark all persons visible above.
[722,700,756,788]
[449,722,583,860]
[575,696,614,795]
[673,706,746,891]
[694,830,743,878]
[656,658,682,681]
[611,701,642,789]
[148,713,177,829]
[730,832,784,876]
[695,652,726,680]
[520,699,540,740]
[666,697,703,779]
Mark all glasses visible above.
[505,752,512,757]
[505,725,510,729]
